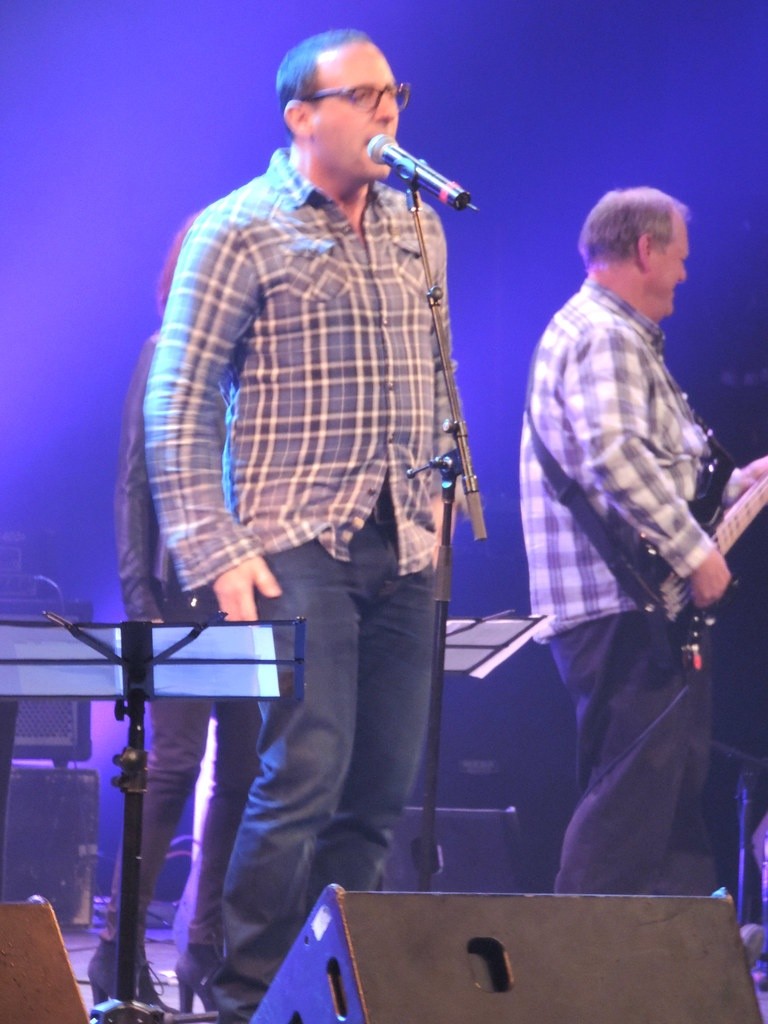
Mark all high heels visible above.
[87,939,179,1014]
[172,946,219,1011]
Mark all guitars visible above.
[624,466,768,689]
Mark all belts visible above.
[365,489,395,526]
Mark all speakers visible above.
[252,883,763,1024]
[374,807,527,892]
[0,763,99,928]
[0,598,94,757]
[0,900,90,1024]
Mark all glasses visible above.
[297,81,411,112]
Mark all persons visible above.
[84,26,451,1024]
[519,188,767,897]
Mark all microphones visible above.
[367,134,481,211]
